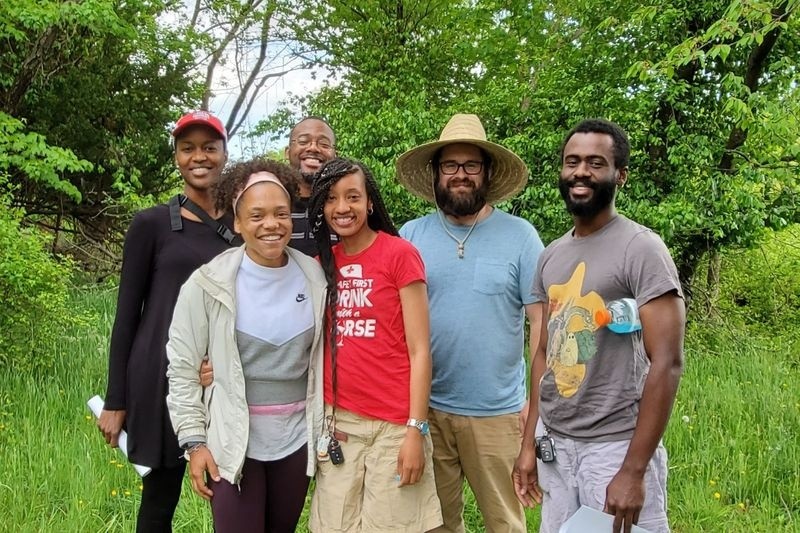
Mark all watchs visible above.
[406,418,430,434]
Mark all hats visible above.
[173,112,228,145]
[396,112,528,205]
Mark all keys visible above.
[327,436,344,464]
[534,432,556,462]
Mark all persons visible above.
[510,120,686,533]
[284,117,337,257]
[397,113,546,533]
[165,157,328,533]
[96,110,244,533]
[199,159,443,533]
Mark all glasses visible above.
[438,159,485,175]
[289,136,337,153]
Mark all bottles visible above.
[594,298,641,334]
